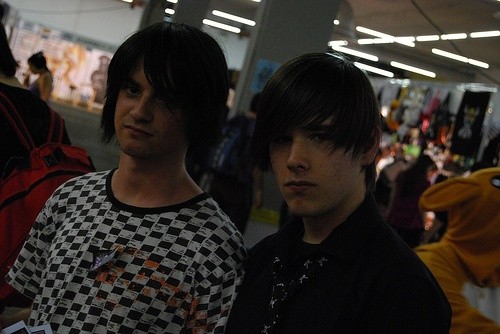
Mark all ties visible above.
[260,254,330,334]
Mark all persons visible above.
[0,0,71,185]
[27,52,53,103]
[200,51,500,334]
[5,20,248,334]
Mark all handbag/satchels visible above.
[199,114,253,177]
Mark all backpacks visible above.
[0,89,97,298]
